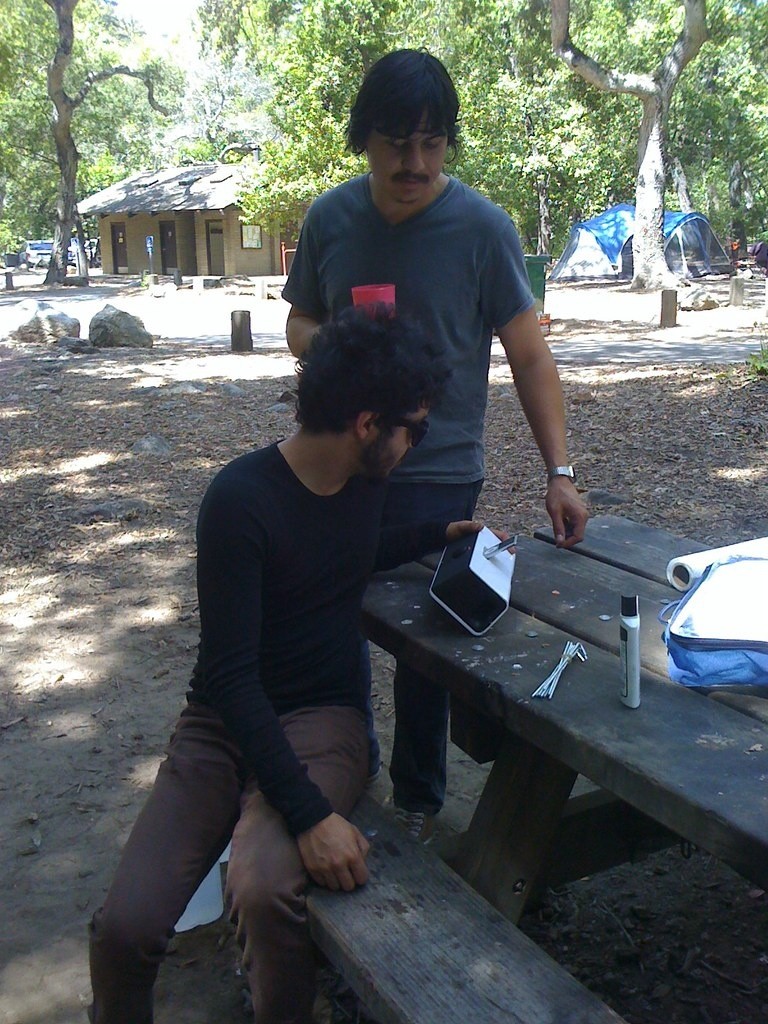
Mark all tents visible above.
[547,203,733,281]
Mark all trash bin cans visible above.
[524,253,552,323]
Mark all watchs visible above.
[547,465,577,486]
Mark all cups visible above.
[351,284,396,322]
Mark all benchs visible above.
[306,791,631,1024]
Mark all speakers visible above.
[429,525,517,637]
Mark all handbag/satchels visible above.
[658,558,768,688]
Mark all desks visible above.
[359,512,768,934]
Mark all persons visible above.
[750,240,768,278]
[86,301,517,1024]
[280,48,589,847]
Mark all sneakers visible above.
[393,807,436,845]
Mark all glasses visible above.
[382,412,429,448]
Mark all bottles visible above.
[619,592,641,709]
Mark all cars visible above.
[17,240,55,266]
[67,236,79,267]
